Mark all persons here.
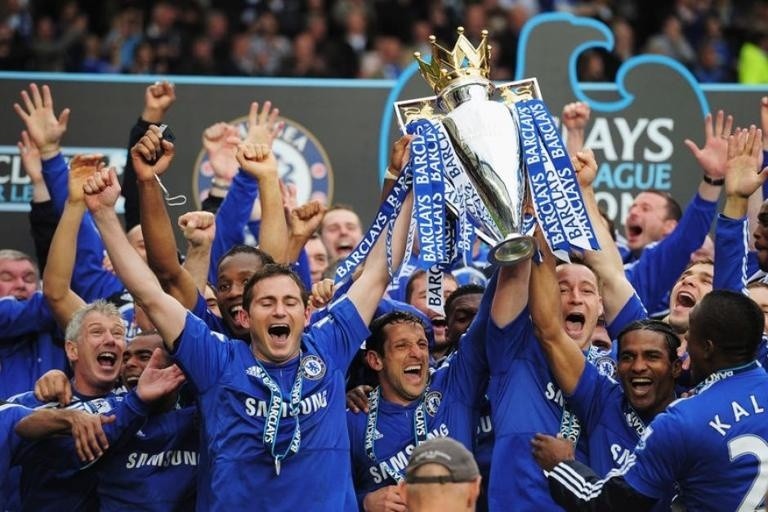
[0,0,767,511]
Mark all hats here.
[405,437,480,484]
